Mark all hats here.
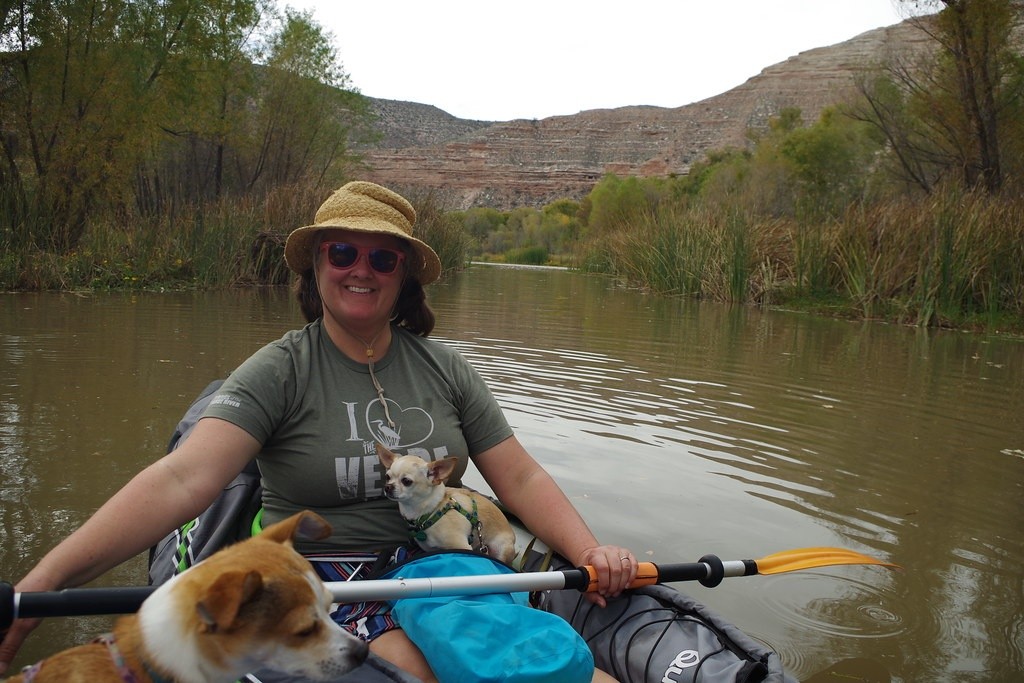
[284,181,441,286]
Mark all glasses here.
[318,242,407,275]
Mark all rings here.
[620,556,629,561]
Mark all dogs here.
[0,510,370,683]
[374,442,516,567]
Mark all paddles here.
[0,548,902,643]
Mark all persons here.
[0,181,639,683]
